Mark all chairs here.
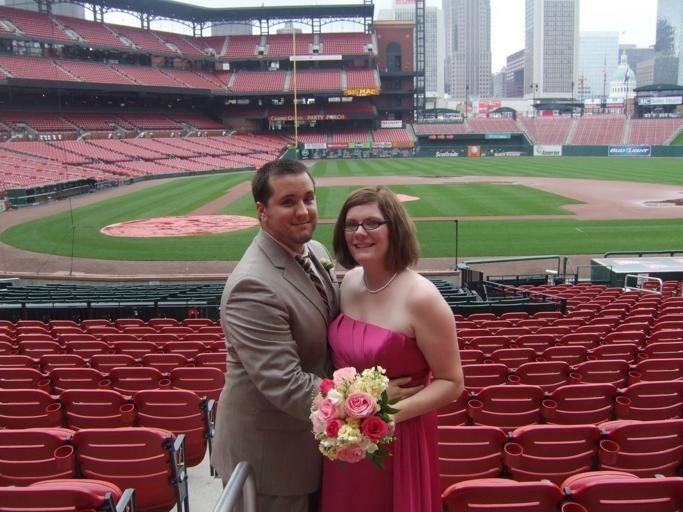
[1,278,682,512]
[0,7,683,202]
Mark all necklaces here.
[361,268,401,293]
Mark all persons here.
[309,184,465,511]
[211,161,423,512]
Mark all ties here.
[302,256,331,315]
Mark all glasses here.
[344,219,388,230]
